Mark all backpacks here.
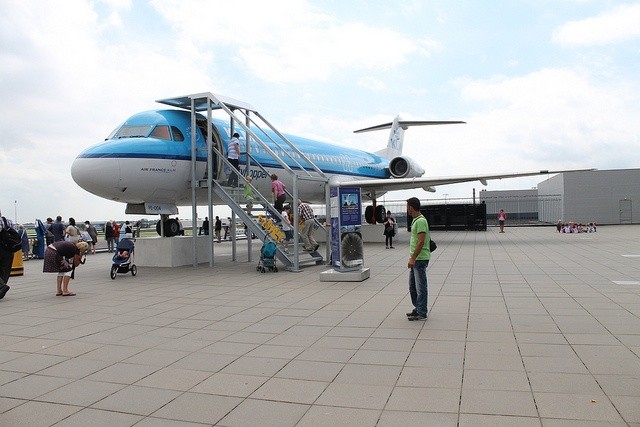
[0,217,22,253]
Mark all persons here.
[0,277,9,298]
[564,223,570,232]
[557,219,562,231]
[44,217,54,247]
[119,250,128,256]
[0,216,22,284]
[570,225,575,232]
[112,221,120,246]
[227,132,240,187]
[50,215,66,242]
[124,221,132,237]
[105,221,114,252]
[215,215,222,242]
[588,222,594,232]
[203,216,209,235]
[562,223,565,232]
[243,221,248,236]
[43,240,90,296]
[406,197,437,320]
[383,209,396,248]
[281,204,291,240]
[132,219,142,236]
[31,240,40,259]
[296,198,320,251]
[577,223,587,232]
[497,207,506,232]
[270,173,287,213]
[227,216,232,234]
[66,216,80,238]
[587,223,596,231]
[35,218,48,258]
[18,224,30,260]
[84,220,97,254]
[243,175,254,215]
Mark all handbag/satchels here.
[430,239,437,252]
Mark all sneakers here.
[407,309,419,316]
[0,285,9,299]
[390,247,395,249]
[314,244,319,251]
[408,314,427,321]
[386,247,389,249]
[303,248,313,251]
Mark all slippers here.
[63,291,76,296]
[56,293,62,296]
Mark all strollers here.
[111,238,137,279]
[257,231,278,273]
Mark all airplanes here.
[71,109,597,238]
